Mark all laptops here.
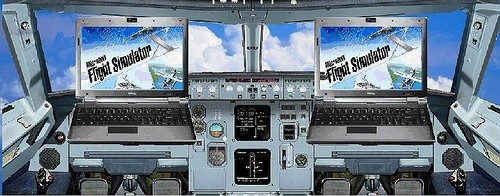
[67,16,197,146]
[305,15,436,146]
